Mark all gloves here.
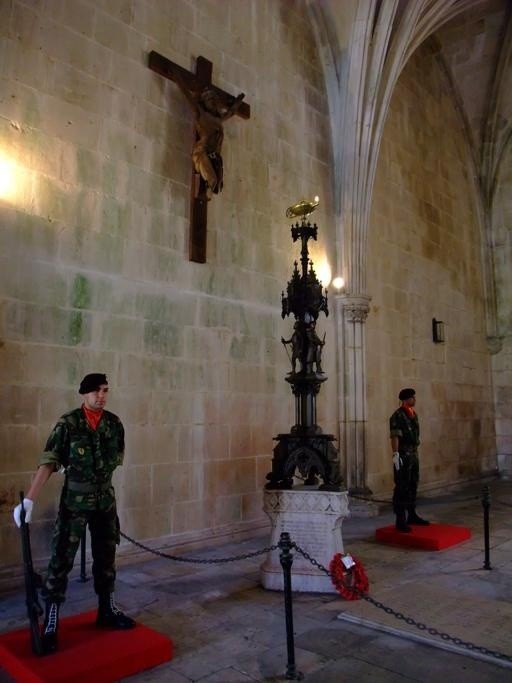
[14,498,34,528]
[392,452,404,470]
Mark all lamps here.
[433,317,445,342]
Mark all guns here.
[393,448,406,530]
[19,490,43,657]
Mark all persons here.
[12,372,137,651]
[389,386,430,532]
[175,72,244,201]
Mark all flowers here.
[329,554,370,602]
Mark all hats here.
[78,374,108,395]
[399,389,415,399]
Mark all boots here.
[395,506,429,533]
[95,592,135,631]
[41,602,60,654]
